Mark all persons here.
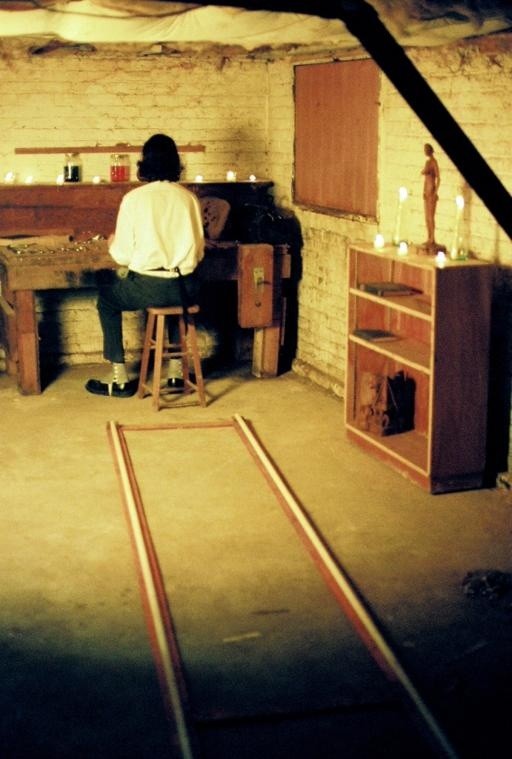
[420,144,440,244]
[86,134,206,397]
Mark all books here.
[354,329,397,343]
[359,281,411,296]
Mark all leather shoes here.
[85,378,132,397]
[168,373,195,396]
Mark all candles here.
[193,170,260,183]
[435,251,445,265]
[2,169,100,186]
[373,233,386,247]
[395,241,408,257]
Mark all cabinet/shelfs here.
[343,238,496,496]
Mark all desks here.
[1,240,294,392]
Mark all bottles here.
[64,150,84,182]
[108,153,132,182]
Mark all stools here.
[138,301,209,409]
